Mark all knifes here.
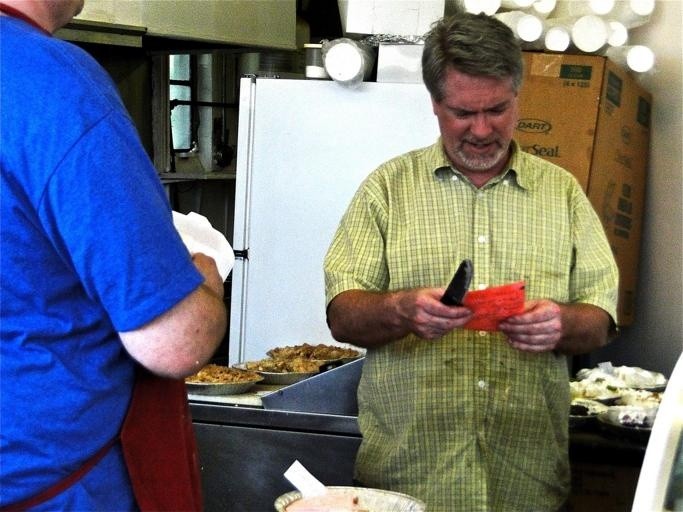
[438,259,473,307]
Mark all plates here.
[274,487,425,512]
[568,368,665,434]
[229,359,313,386]
[264,350,360,362]
[184,382,254,398]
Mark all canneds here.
[303,44,327,79]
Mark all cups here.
[301,43,328,79]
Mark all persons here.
[323,11,622,512]
[0,2,240,511]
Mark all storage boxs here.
[510,51,653,330]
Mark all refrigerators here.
[222,78,440,364]
